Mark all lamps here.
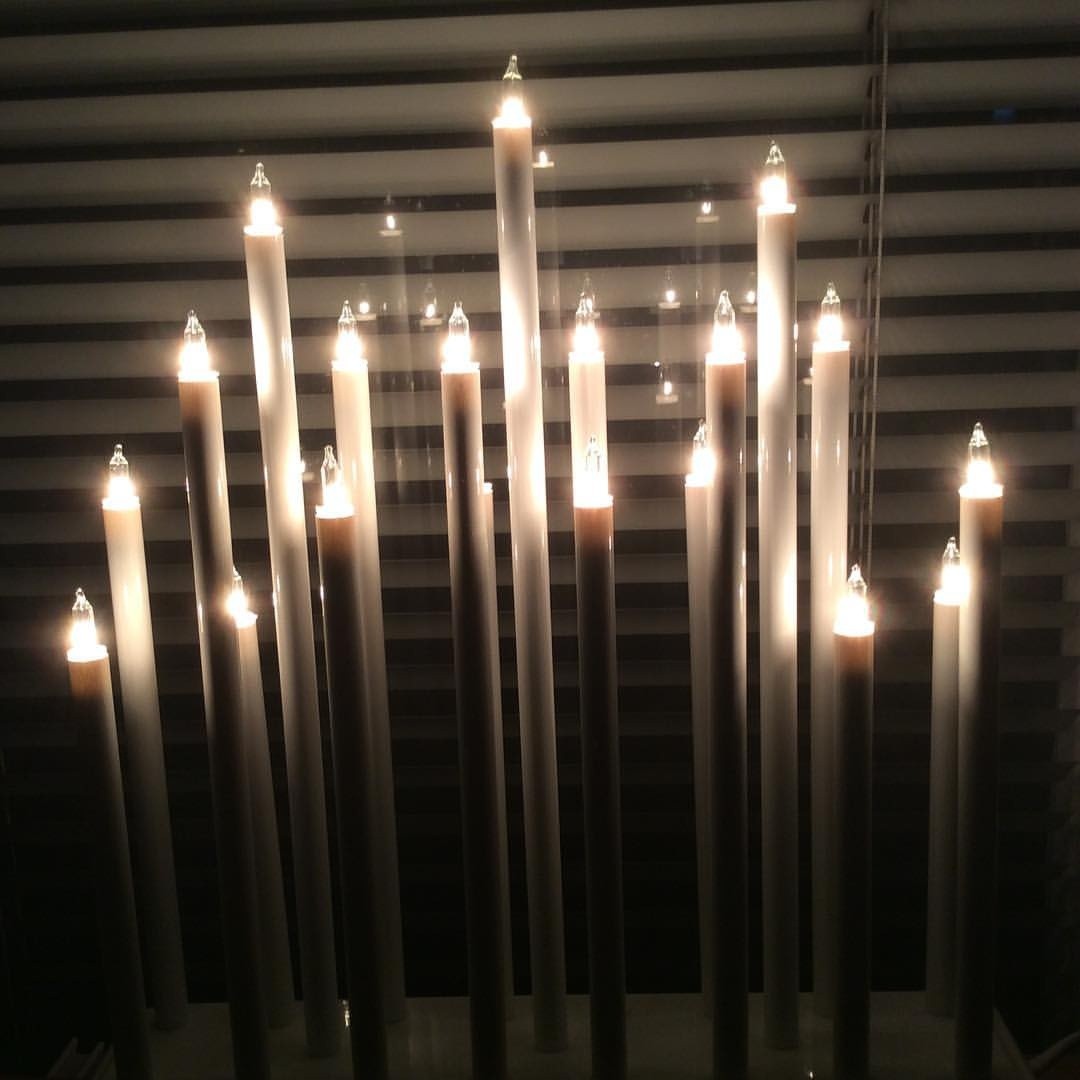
[64,53,1003,1080]
